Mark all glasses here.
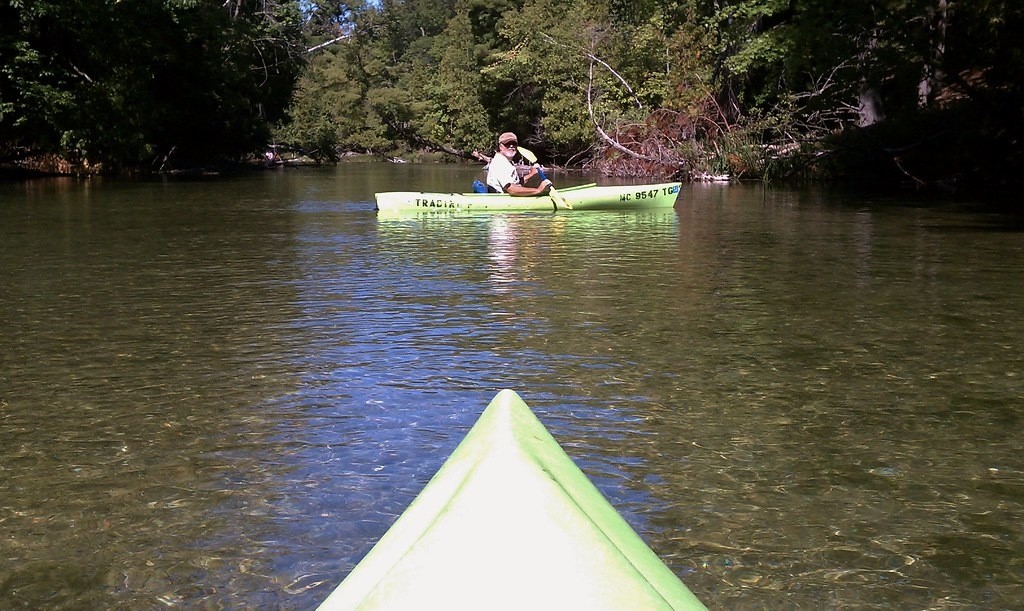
[503,142,516,147]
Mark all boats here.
[373,182,684,214]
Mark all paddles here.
[517,145,573,210]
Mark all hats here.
[498,131,518,146]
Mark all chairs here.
[473,180,487,193]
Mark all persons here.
[486,132,553,197]
